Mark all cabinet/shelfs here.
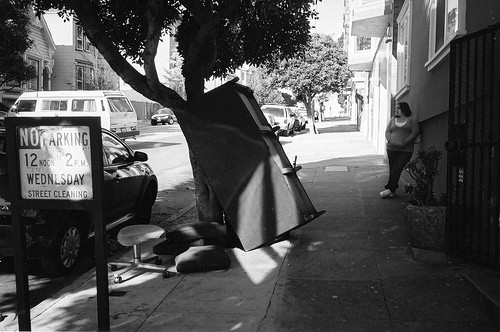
[175,76,327,252]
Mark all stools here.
[108,224,180,283]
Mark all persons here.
[380,101,421,197]
[320,102,326,122]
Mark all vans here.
[6,91,141,140]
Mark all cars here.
[259,103,310,139]
[0,129,159,276]
[151,108,175,126]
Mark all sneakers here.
[389,192,396,198]
[379,189,391,197]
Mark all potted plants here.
[403,148,446,250]
